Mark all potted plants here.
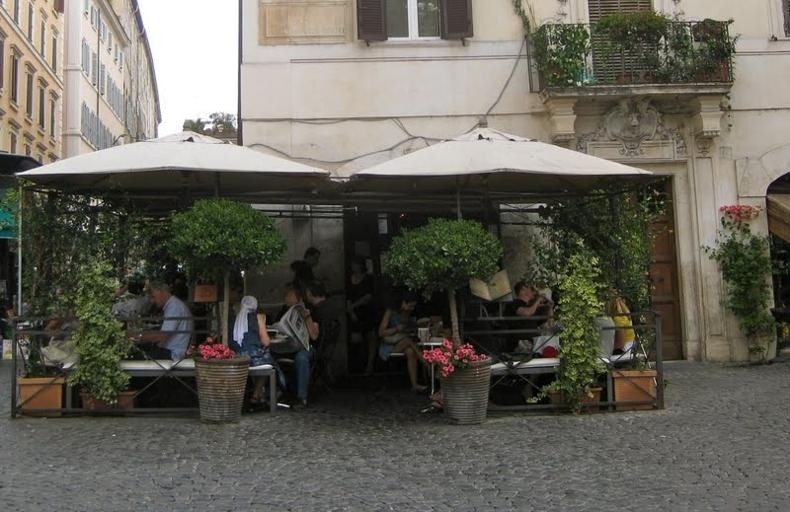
[526,227,603,413]
[378,216,500,425]
[13,188,73,419]
[547,185,658,411]
[69,255,137,412]
[166,196,287,423]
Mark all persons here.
[41,247,346,415]
[346,260,635,415]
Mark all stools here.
[389,352,406,356]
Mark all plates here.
[271,335,289,344]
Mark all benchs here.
[490,353,644,411]
[63,358,278,418]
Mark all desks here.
[270,335,288,346]
[468,314,546,351]
[126,330,165,360]
[417,336,450,415]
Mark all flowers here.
[182,342,233,361]
[423,339,486,379]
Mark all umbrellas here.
[12,129,330,204]
[350,127,651,226]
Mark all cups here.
[417,327,431,343]
